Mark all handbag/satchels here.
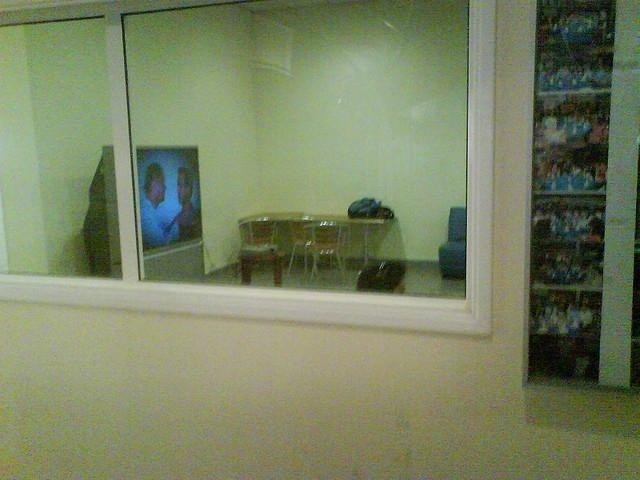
[348,199,394,219]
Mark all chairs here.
[236,215,349,289]
[437,206,467,280]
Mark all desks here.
[238,212,387,269]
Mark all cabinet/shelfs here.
[529,1,640,395]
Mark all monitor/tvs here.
[81,145,205,266]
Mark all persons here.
[169,167,199,236]
[355,262,408,296]
[136,163,180,245]
